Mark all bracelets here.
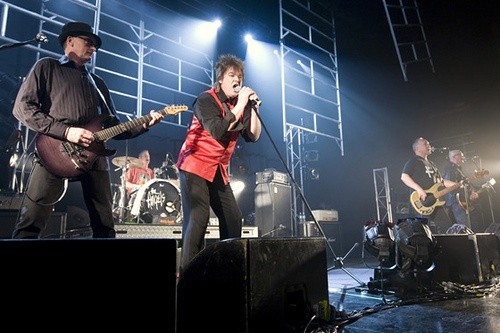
[64,126,70,139]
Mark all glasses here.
[78,36,99,52]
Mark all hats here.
[58,21,103,49]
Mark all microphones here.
[35,32,49,44]
[164,153,169,166]
[235,86,263,106]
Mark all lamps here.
[363,220,436,274]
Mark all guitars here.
[34,103,189,179]
[410,169,489,216]
[457,178,496,212]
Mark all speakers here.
[175,237,327,332]
[408,232,500,286]
[0,238,176,333]
[254,183,293,237]
[0,210,68,239]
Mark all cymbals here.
[111,157,144,168]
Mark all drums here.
[156,166,180,188]
[137,181,182,225]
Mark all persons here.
[441,149,478,230]
[13,22,164,239]
[401,137,467,234]
[125,151,154,223]
[176,55,262,277]
